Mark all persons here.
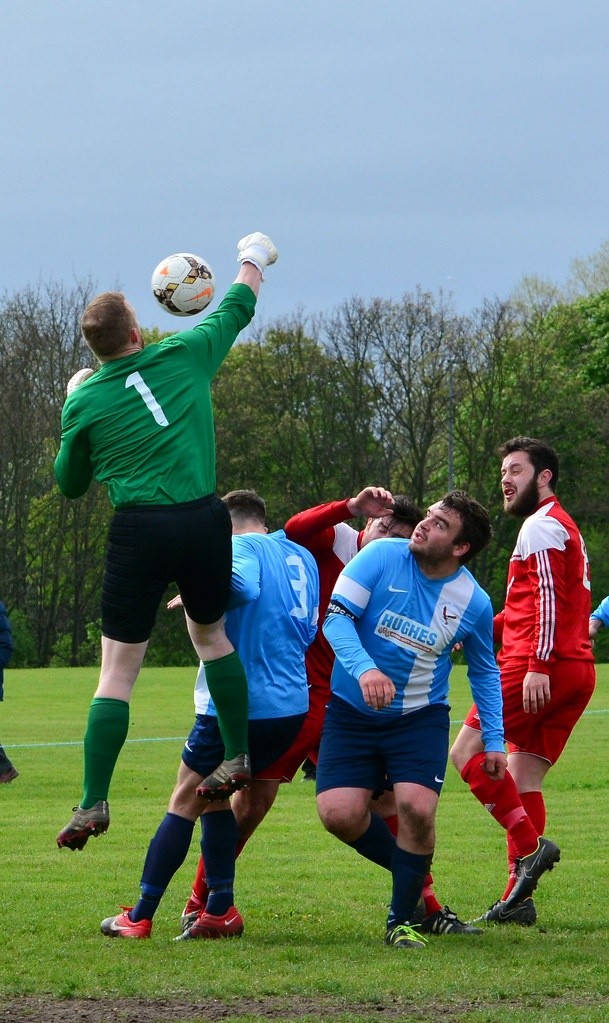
[313,490,506,949]
[447,437,597,924]
[178,485,490,937]
[53,229,281,851]
[94,490,318,937]
[0,600,19,785]
[588,596,609,646]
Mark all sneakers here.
[56,801,109,853]
[181,905,204,933]
[384,924,428,951]
[100,906,154,941]
[0,767,19,783]
[465,893,537,925]
[413,905,485,937]
[170,906,244,940]
[195,755,253,799]
[504,836,561,908]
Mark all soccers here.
[152,252,215,316]
